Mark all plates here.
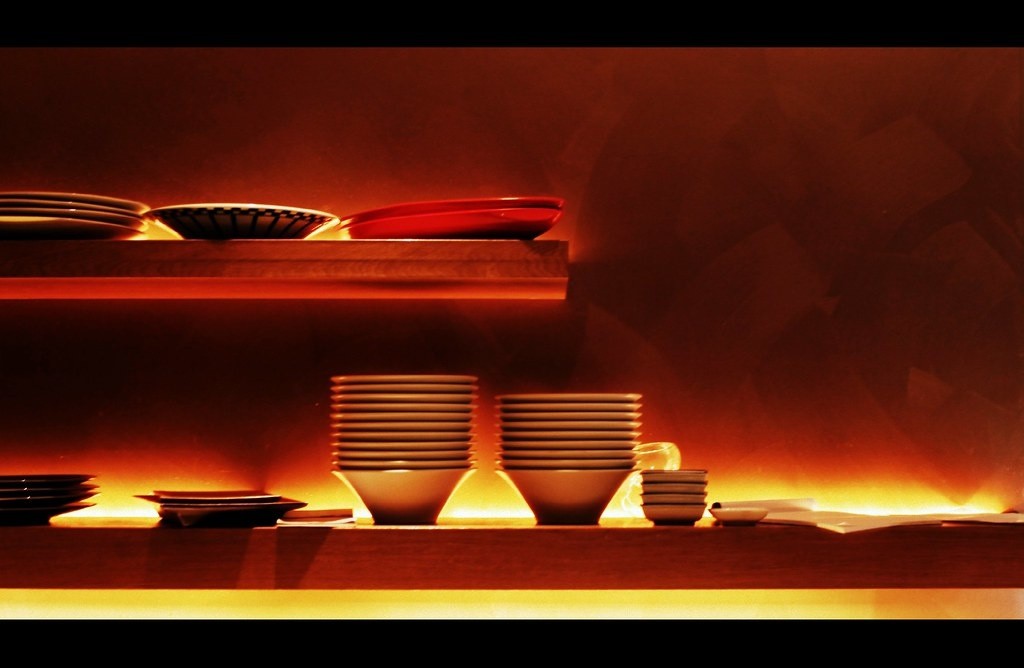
[329,375,480,525]
[0,189,149,240]
[140,202,340,239]
[708,508,769,526]
[338,196,566,239]
[0,472,102,527]
[133,491,308,528]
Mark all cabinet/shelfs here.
[1,236,1024,588]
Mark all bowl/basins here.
[493,394,642,526]
[640,470,708,526]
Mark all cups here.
[634,442,681,485]
